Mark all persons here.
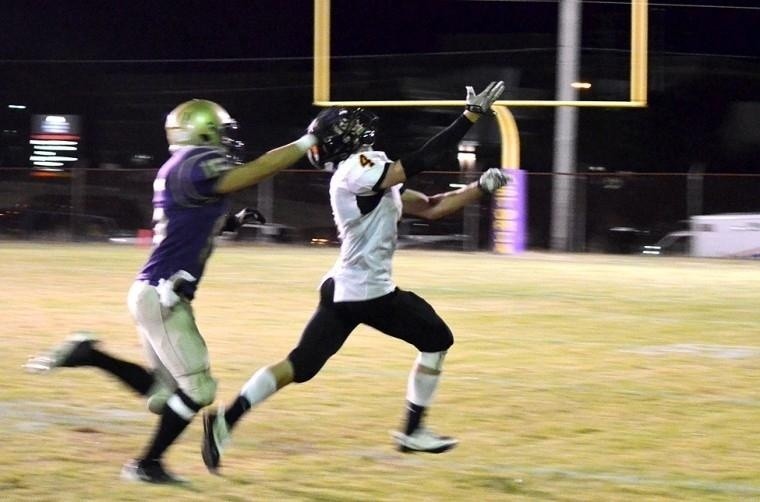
[18,97,348,492]
[200,79,510,475]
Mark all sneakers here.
[389,428,458,453]
[202,403,227,469]
[23,333,101,374]
[122,454,190,484]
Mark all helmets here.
[164,98,246,165]
[307,106,380,172]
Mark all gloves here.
[479,168,512,195]
[223,207,265,231]
[465,80,506,116]
[307,107,348,146]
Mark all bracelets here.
[294,131,321,155]
